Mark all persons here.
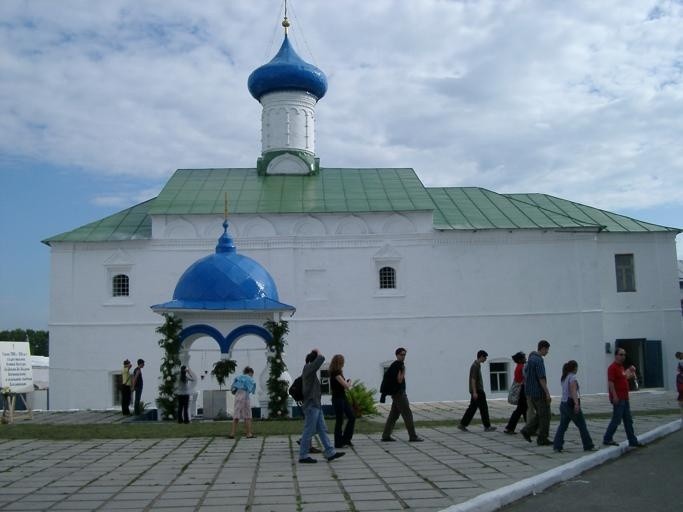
[174,365,197,424]
[298,348,346,463]
[504,351,527,434]
[520,340,553,446]
[121,359,133,416]
[329,354,356,448]
[381,348,424,441]
[296,354,322,453]
[603,347,647,448]
[675,352,683,402]
[131,359,145,416]
[553,360,600,453]
[226,366,256,438]
[457,350,497,432]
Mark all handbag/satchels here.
[289,376,305,402]
[508,382,522,406]
[567,397,581,408]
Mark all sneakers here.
[484,427,496,432]
[590,446,601,452]
[457,423,469,432]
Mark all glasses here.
[618,354,628,357]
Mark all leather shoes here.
[310,447,322,454]
[603,440,618,446]
[629,442,647,448]
[520,430,533,443]
[299,458,318,464]
[329,452,346,461]
[537,439,554,446]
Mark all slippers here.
[381,437,397,441]
[409,438,424,441]
[504,429,517,434]
